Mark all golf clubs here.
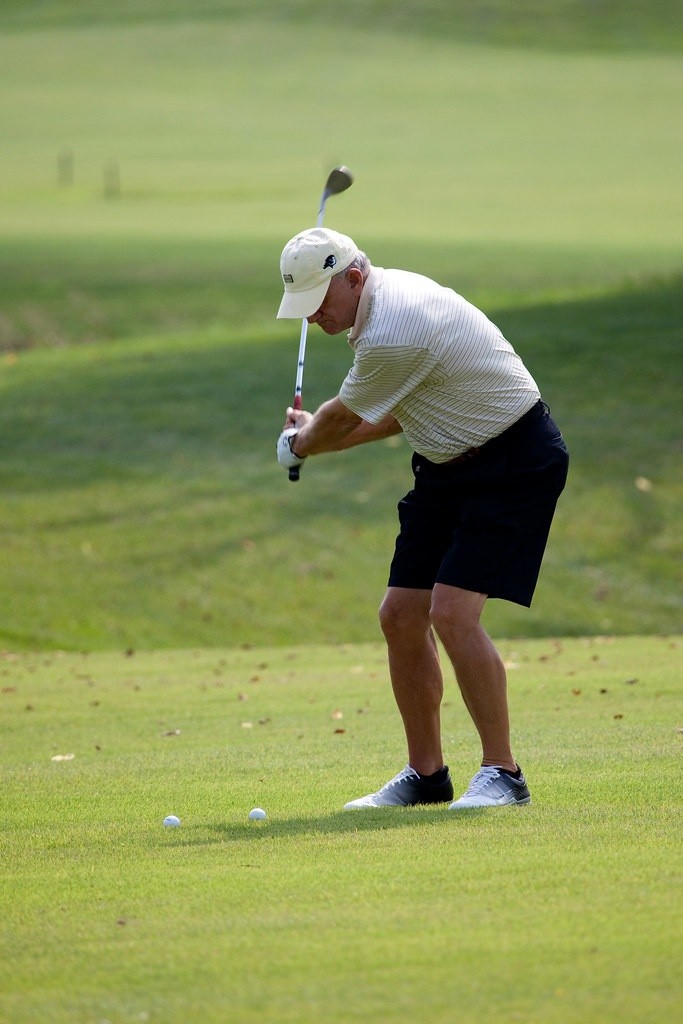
[288,166,355,482]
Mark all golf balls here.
[163,815,180,828]
[249,808,266,819]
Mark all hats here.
[276,225,359,319]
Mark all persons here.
[276,229,570,814]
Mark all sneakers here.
[343,763,454,810]
[448,763,532,808]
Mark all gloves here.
[276,428,308,469]
